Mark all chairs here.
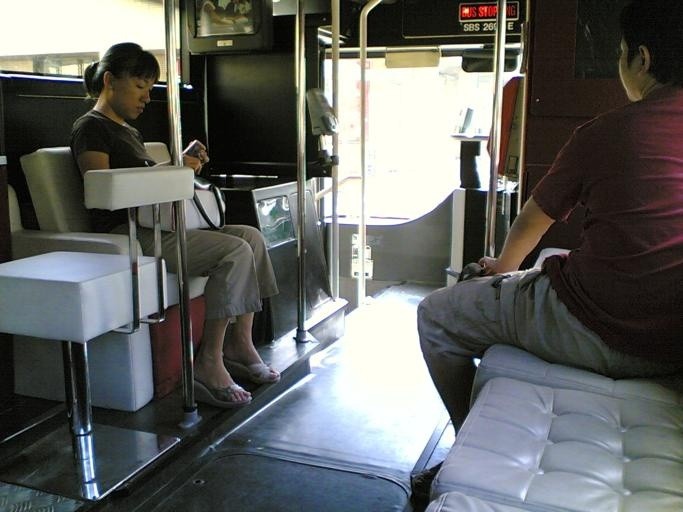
[9,145,211,412]
[425,248,683,512]
[0,165,195,503]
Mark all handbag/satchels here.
[138,157,227,231]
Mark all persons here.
[418,1,683,436]
[70,42,281,409]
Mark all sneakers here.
[409,456,448,499]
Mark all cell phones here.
[182,140,207,160]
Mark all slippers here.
[221,352,282,386]
[181,373,254,409]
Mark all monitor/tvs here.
[187,0,274,55]
